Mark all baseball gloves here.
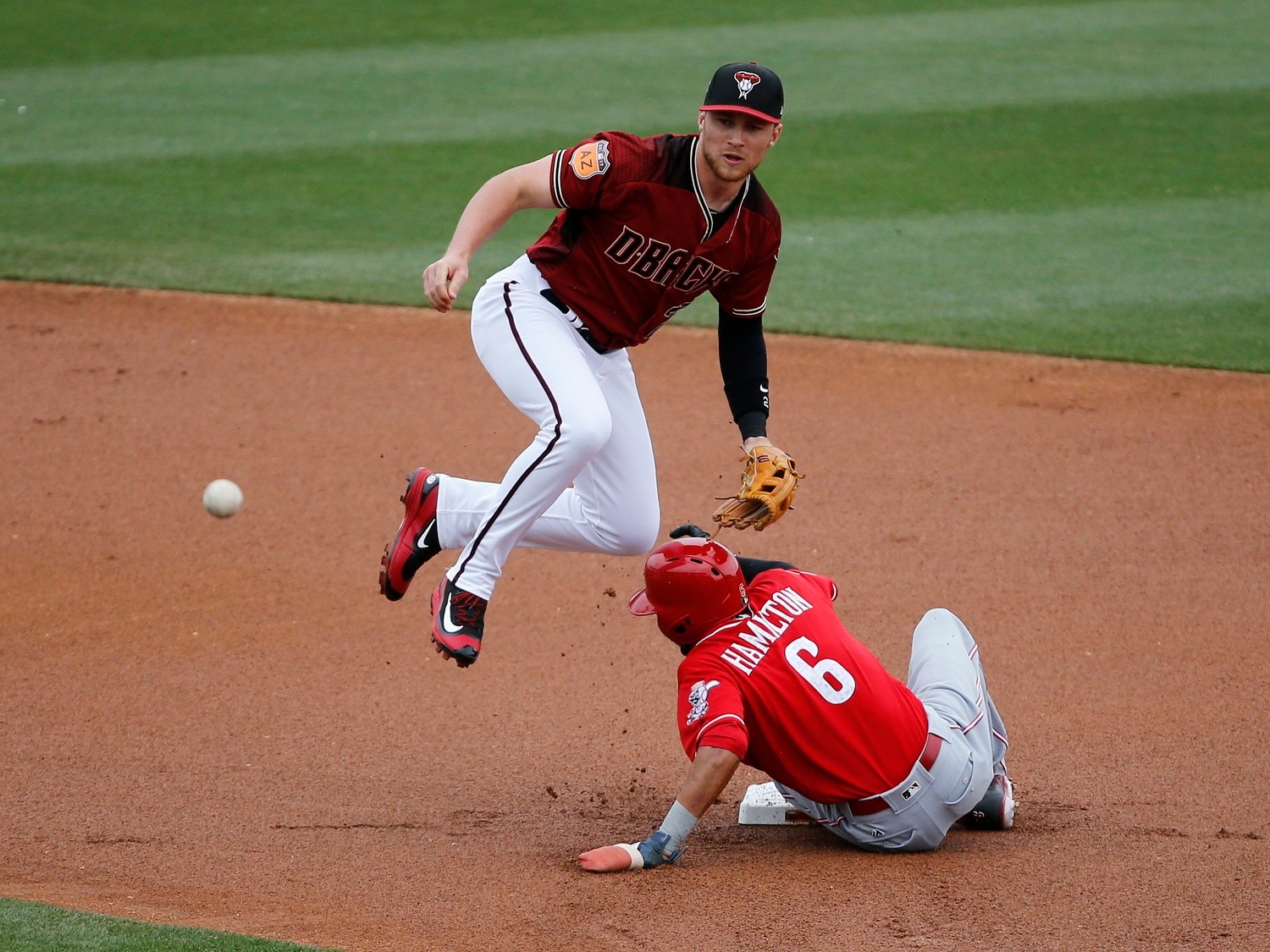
[705,444,806,544]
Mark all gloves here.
[579,841,665,874]
[668,526,711,542]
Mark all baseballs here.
[201,479,244,520]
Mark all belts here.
[541,289,617,355]
[837,732,941,819]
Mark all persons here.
[578,524,1015,872]
[379,61,797,669]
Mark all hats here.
[700,61,786,125]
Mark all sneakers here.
[377,466,442,602]
[954,772,1013,832]
[430,576,488,669]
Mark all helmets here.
[629,535,748,645]
